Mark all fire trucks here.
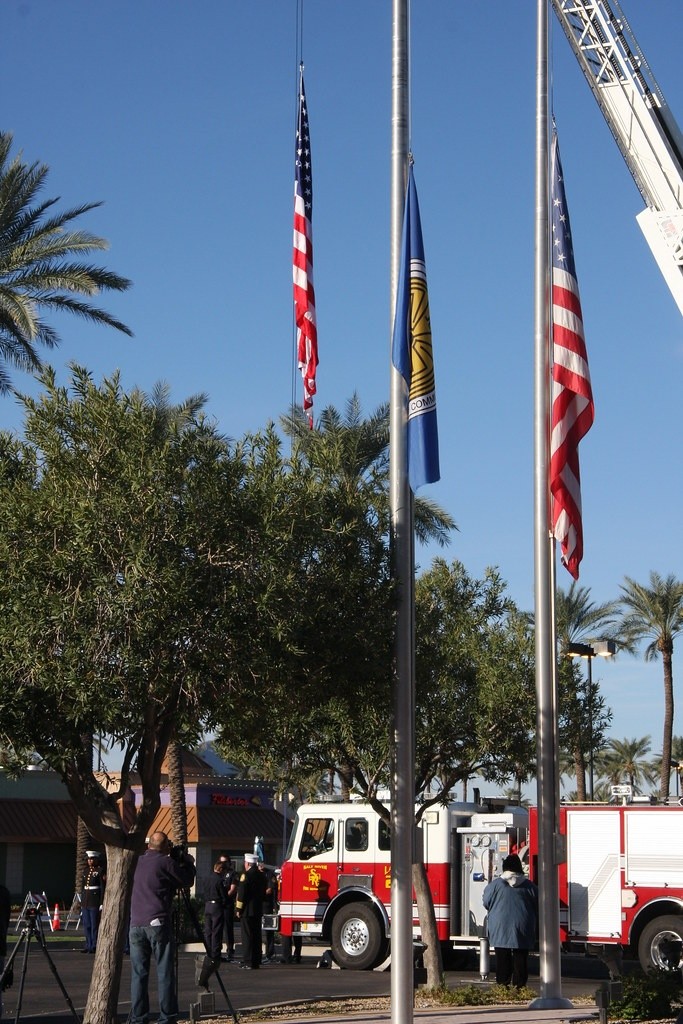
[262,0,683,984]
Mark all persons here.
[257,865,278,965]
[219,854,237,962]
[129,832,195,1024]
[238,853,265,970]
[302,835,334,854]
[482,854,538,991]
[80,851,104,953]
[276,935,302,964]
[196,861,228,990]
[352,823,368,851]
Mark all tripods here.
[0,908,82,1024]
[174,886,240,1024]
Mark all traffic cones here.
[49,904,64,931]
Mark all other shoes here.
[80,949,96,954]
[224,954,235,961]
[276,958,290,965]
[261,957,273,964]
[237,964,252,969]
[291,956,302,964]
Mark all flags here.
[391,156,441,498]
[292,66,321,432]
[549,127,596,582]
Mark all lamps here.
[413,939,429,969]
[193,953,221,991]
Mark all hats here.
[244,853,259,864]
[85,850,101,858]
[503,854,523,874]
